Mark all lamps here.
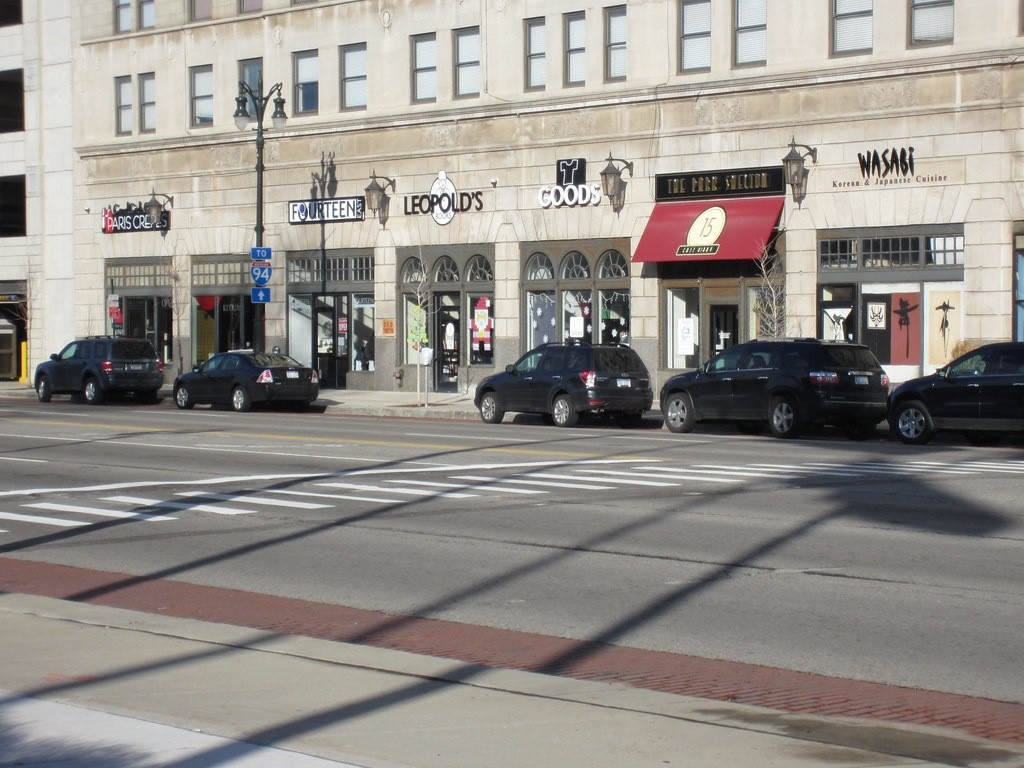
[146,186,174,231]
[781,134,817,187]
[364,169,396,219]
[600,149,633,202]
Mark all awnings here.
[630,196,784,262]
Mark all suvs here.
[35,335,163,405]
[473,342,653,427]
[660,335,889,439]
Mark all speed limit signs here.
[251,262,273,286]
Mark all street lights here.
[234,70,288,353]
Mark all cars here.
[888,341,1024,445]
[173,350,318,412]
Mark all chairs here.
[999,358,1019,374]
[751,355,766,369]
